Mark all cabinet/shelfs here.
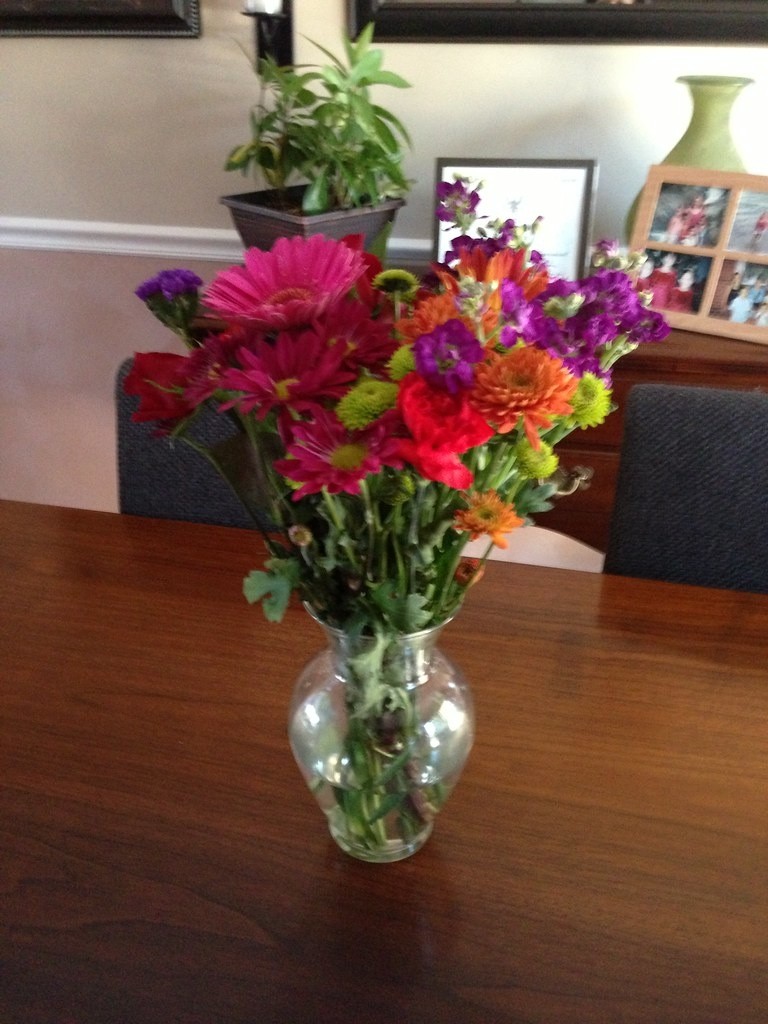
[528,319,768,551]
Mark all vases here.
[624,74,755,244]
[281,601,479,861]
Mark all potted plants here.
[216,25,413,261]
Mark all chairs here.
[604,386,768,589]
[116,354,289,529]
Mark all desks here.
[0,501,768,1022]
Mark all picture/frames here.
[347,0,768,40]
[609,165,768,346]
[0,0,203,40]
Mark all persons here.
[634,194,768,328]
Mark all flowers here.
[117,174,678,653]
